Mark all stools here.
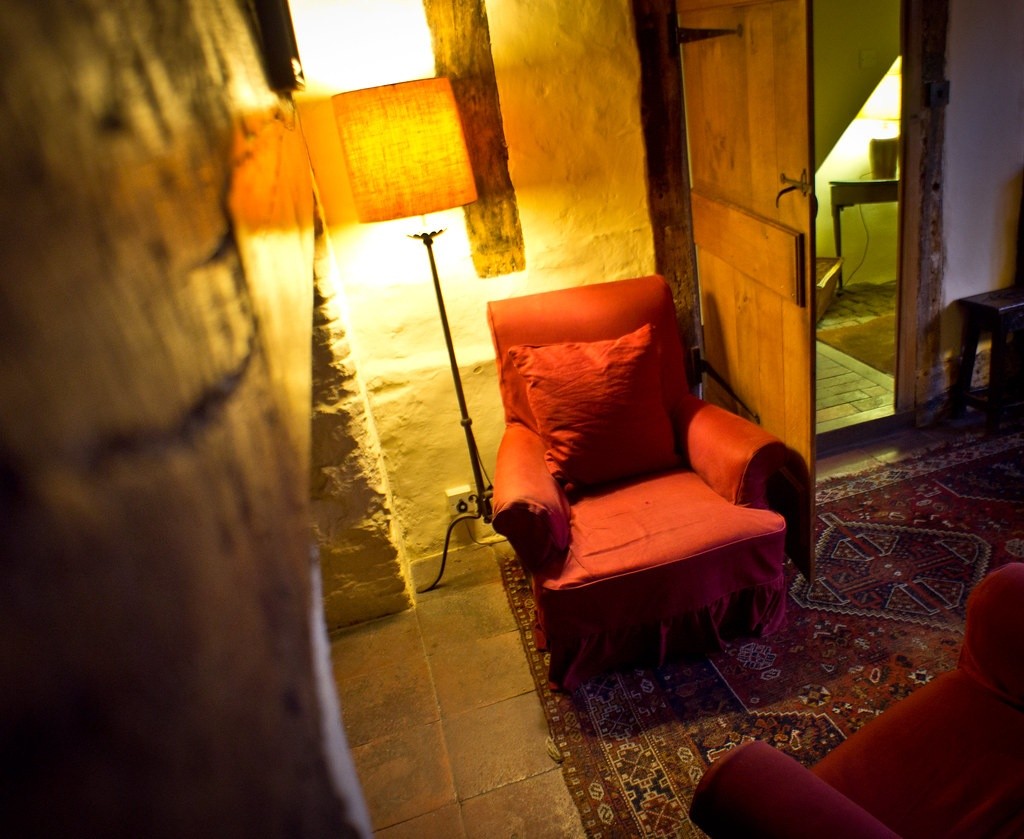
[948,285,1024,417]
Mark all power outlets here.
[445,484,477,517]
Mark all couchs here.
[684,563,1024,839]
[487,274,810,708]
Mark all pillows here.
[506,322,678,495]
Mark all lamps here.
[865,72,902,177]
[332,77,510,596]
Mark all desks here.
[829,177,902,291]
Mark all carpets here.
[496,422,1024,839]
